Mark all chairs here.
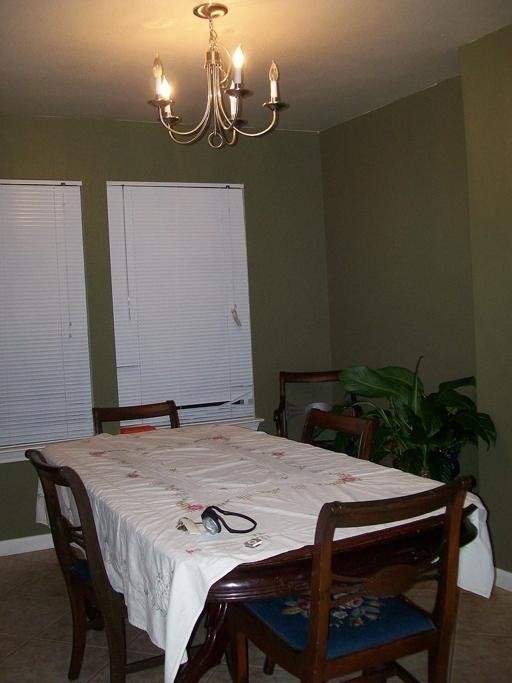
[300,407,374,461]
[25,447,213,681]
[238,474,473,680]
[90,399,181,436]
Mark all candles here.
[147,1,287,148]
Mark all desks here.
[31,422,496,682]
